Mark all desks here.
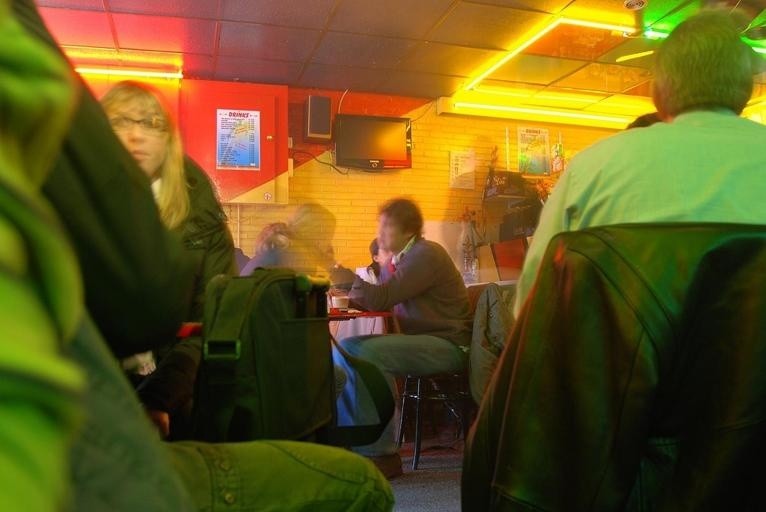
[330,309,393,321]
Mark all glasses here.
[111,117,163,132]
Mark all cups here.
[332,296,349,312]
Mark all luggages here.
[194,267,331,442]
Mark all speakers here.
[303,95,333,140]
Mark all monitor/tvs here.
[335,113,412,172]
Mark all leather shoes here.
[369,454,403,479]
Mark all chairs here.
[393,282,499,472]
[651,236,766,512]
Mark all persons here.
[1,0,766,512]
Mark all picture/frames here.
[518,127,551,177]
[448,150,476,190]
